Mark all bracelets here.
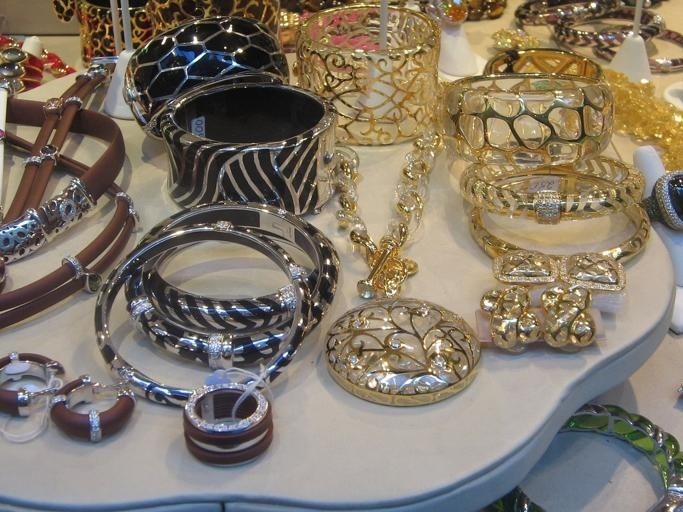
[2,3,682,512]
